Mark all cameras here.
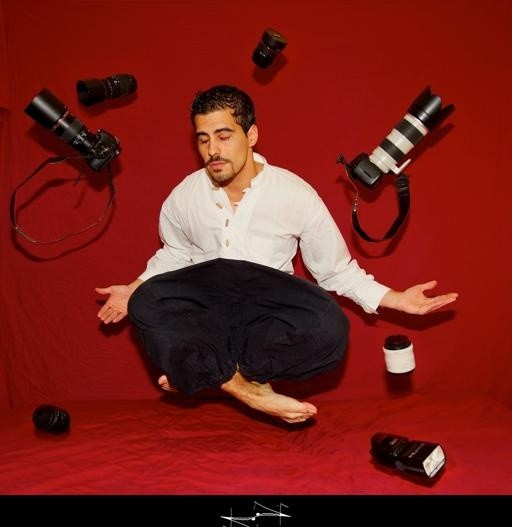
[24,88,121,171]
[349,85,455,189]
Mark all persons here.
[95,86,459,426]
